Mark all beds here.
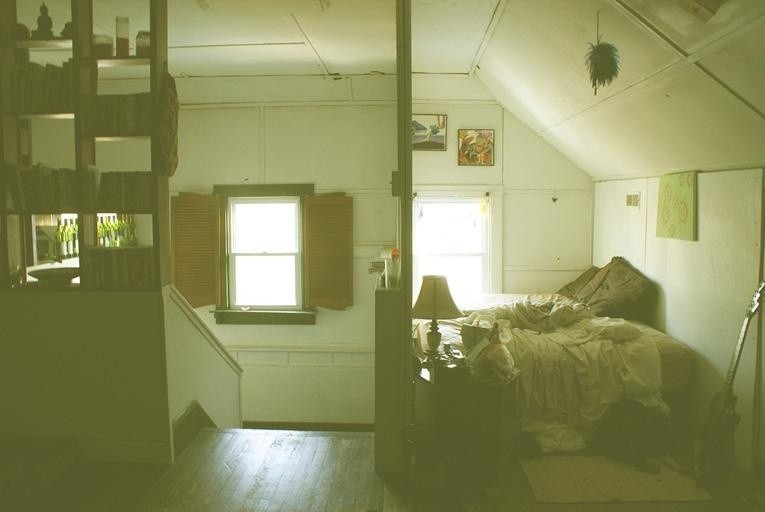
[412,287,696,445]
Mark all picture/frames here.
[457,127,496,168]
[411,113,450,152]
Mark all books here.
[88,170,152,210]
[7,58,73,111]
[6,161,76,210]
[93,92,150,132]
[85,244,155,290]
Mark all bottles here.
[94,16,150,59]
[55,212,136,258]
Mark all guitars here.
[687,281,765,500]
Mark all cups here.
[385,260,400,288]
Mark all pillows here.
[556,258,658,317]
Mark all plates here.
[28,268,80,284]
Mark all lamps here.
[409,276,469,366]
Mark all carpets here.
[520,455,714,504]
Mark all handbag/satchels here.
[462,316,498,347]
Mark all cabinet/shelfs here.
[375,274,407,473]
[0,0,174,291]
